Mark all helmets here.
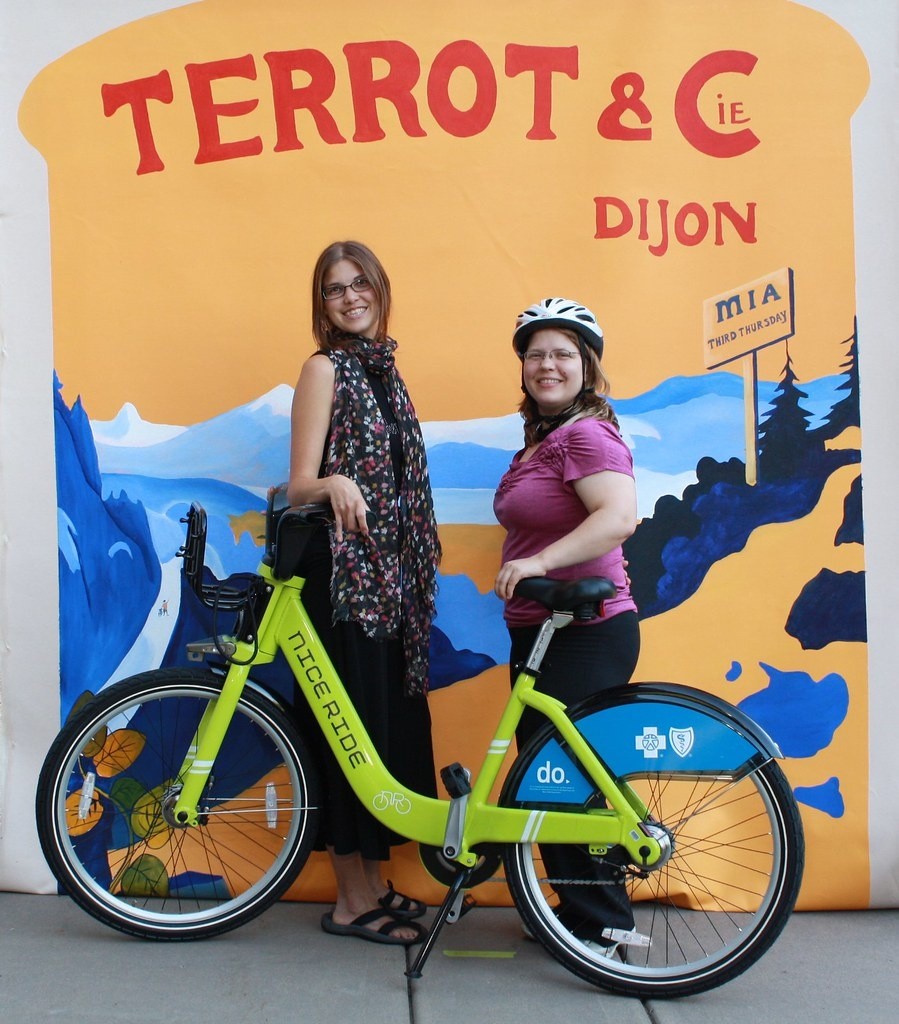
[510,297,604,359]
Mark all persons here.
[494,303,642,959]
[286,241,436,943]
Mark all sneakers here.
[580,923,636,958]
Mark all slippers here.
[319,877,430,946]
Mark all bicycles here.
[31,485,806,1002]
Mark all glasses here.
[521,348,581,361]
[321,278,373,300]
